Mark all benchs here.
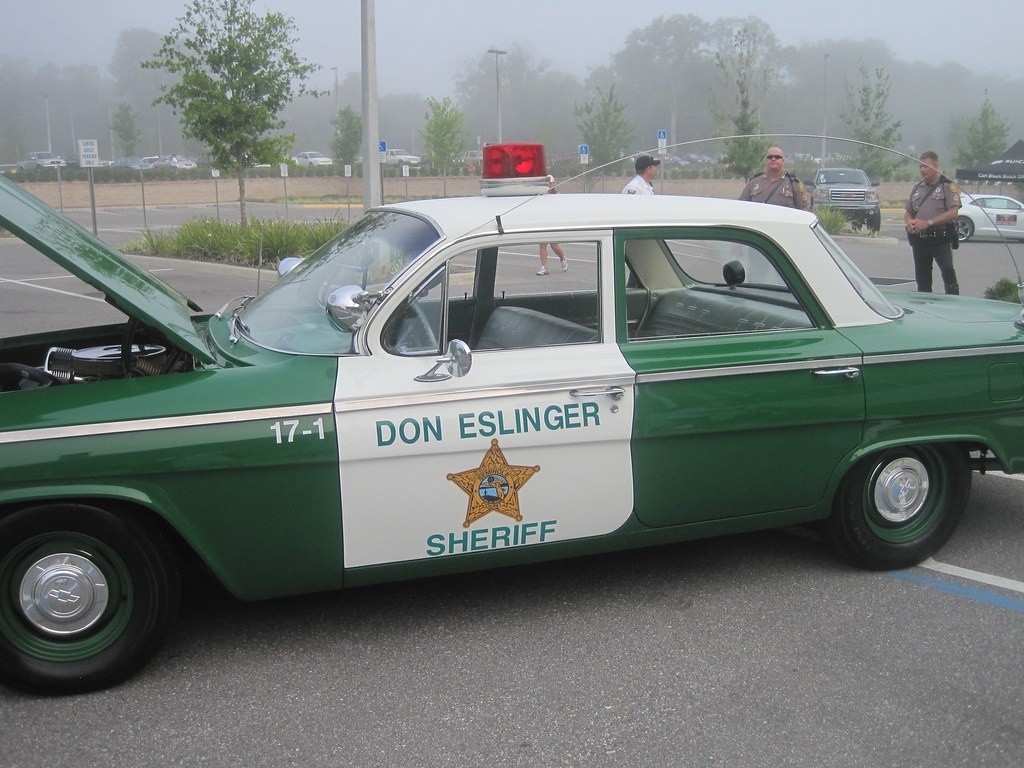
[637,287,816,337]
[475,306,599,347]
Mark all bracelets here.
[928,219,933,225]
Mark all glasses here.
[767,154,783,160]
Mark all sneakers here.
[560,256,568,271]
[536,266,550,275]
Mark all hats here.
[548,174,555,184]
[635,155,661,170]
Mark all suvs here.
[797,166,881,235]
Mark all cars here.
[952,194,1024,240]
[0,140,857,190]
[0,176,1024,691]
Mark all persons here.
[904,150,962,297]
[736,146,808,212]
[622,152,660,288]
[537,174,569,275]
